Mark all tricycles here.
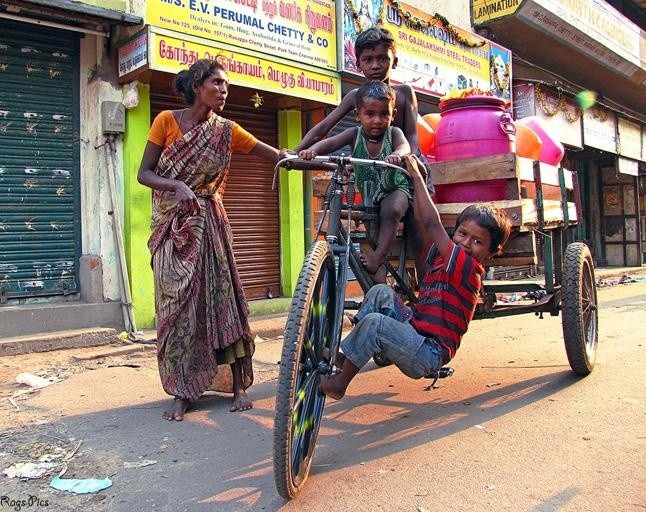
[268,84,600,500]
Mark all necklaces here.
[363,132,385,144]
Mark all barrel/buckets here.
[417,95,565,203]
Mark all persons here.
[353,0,373,34]
[317,153,513,401]
[134,57,357,423]
[296,79,414,286]
[277,25,437,292]
